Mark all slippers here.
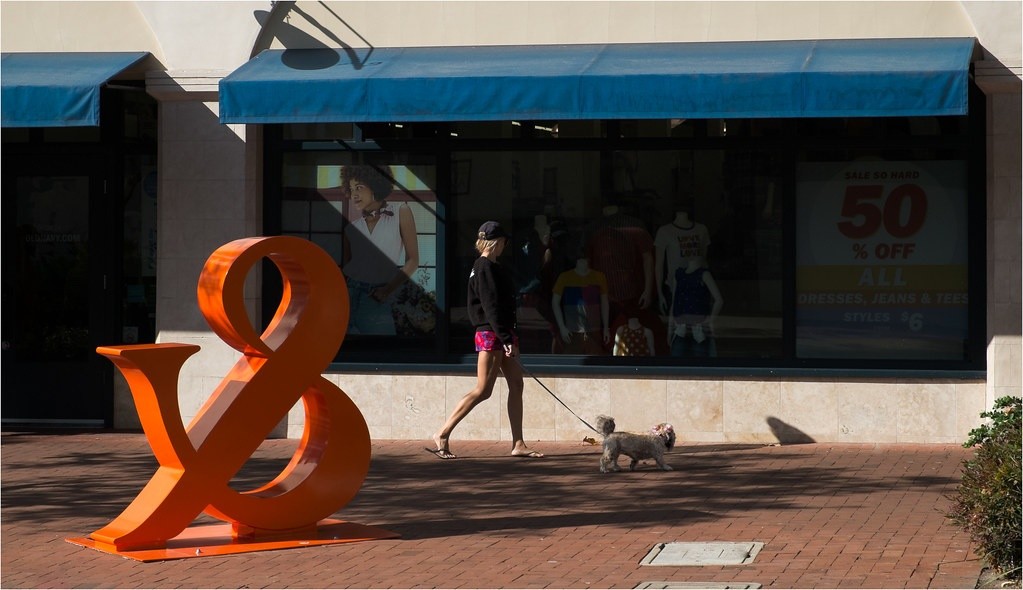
[513,451,544,458]
[425,447,449,459]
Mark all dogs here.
[595,414,676,474]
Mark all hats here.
[477,221,511,240]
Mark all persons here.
[333,150,419,334]
[552,260,611,354]
[433,221,546,458]
[537,228,555,292]
[587,203,726,357]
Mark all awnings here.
[1,52,150,127]
[219,35,981,126]
